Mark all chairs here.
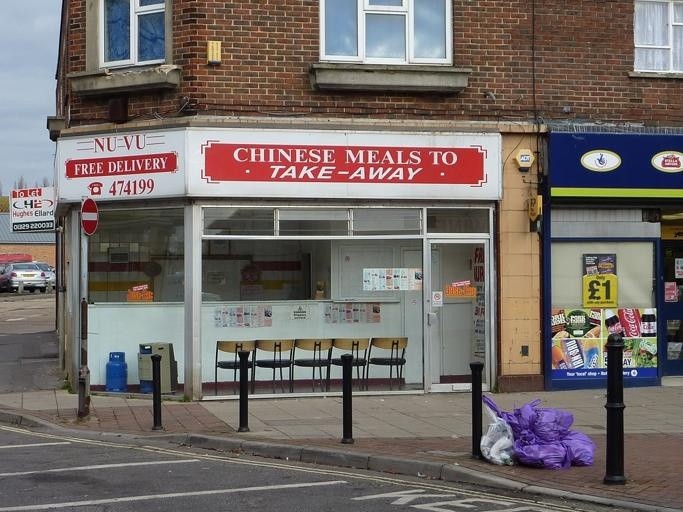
[213,335,411,394]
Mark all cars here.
[0,262,47,295]
[33,260,56,291]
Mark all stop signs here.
[80,196,99,237]
